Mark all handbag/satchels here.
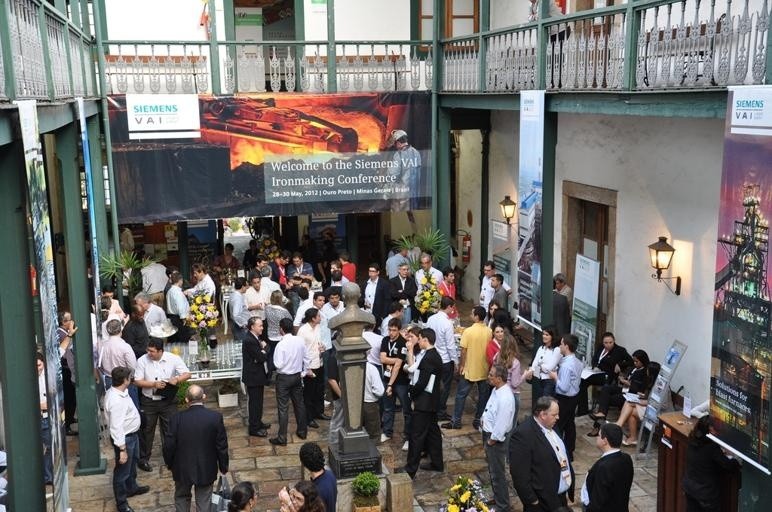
[211,476,232,512]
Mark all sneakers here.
[380,433,409,451]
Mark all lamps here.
[648,237,681,296]
[500,195,517,225]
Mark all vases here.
[351,471,384,512]
[198,348,211,369]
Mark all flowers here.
[184,289,219,344]
[439,475,496,512]
[414,268,440,317]
[258,237,282,267]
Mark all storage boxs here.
[218,389,238,407]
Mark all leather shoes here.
[118,502,134,512]
[269,438,286,446]
[296,414,331,439]
[127,486,149,497]
[420,463,443,472]
[65,418,78,435]
[622,440,637,445]
[138,461,152,471]
[249,424,270,437]
[587,414,605,437]
[438,414,461,429]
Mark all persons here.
[691,401,709,419]
[386,130,421,211]
[0,232,676,511]
[681,416,743,512]
